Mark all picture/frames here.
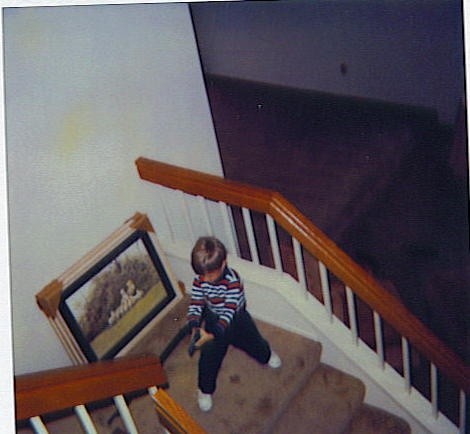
[35,212,186,366]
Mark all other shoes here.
[198,389,212,411]
[268,351,281,369]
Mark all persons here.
[187,236,282,412]
